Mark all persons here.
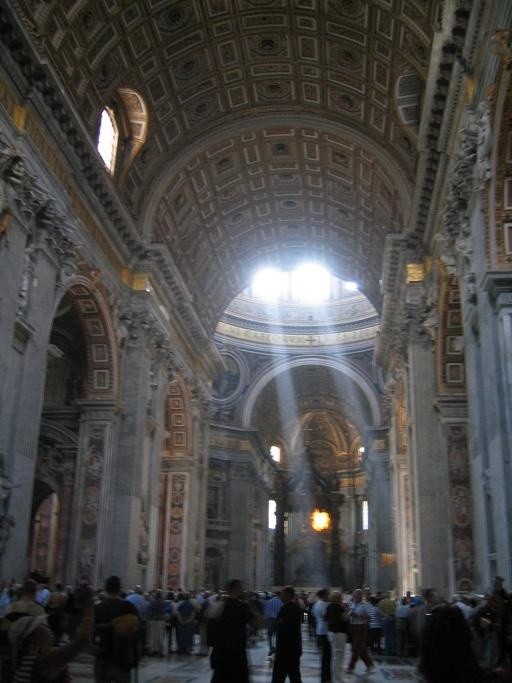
[0,572,512,683]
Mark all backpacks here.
[107,614,147,668]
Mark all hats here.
[8,613,51,646]
